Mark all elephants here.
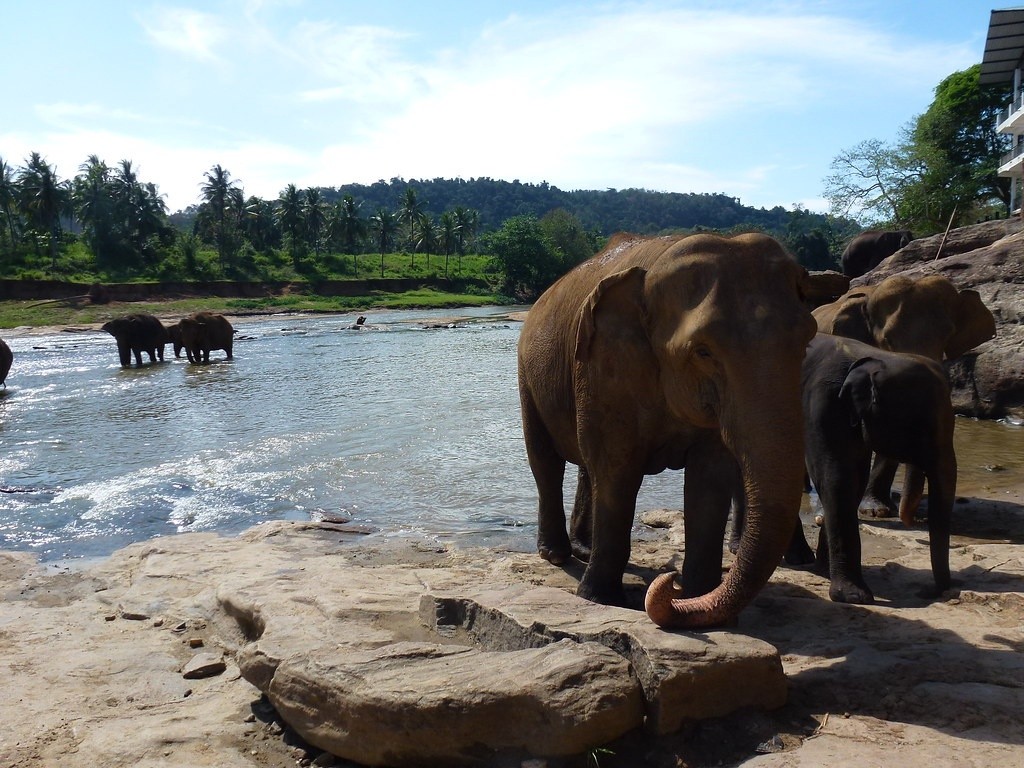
[0,337,14,390]
[100,310,239,367]
[515,227,996,629]
